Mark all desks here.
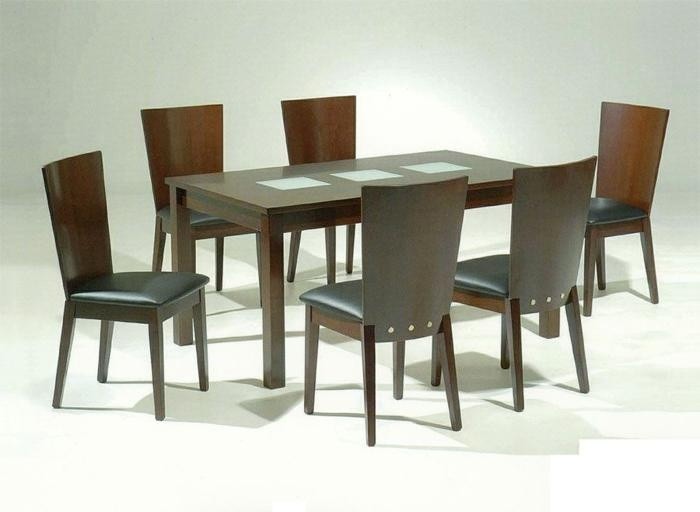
[165,149,537,390]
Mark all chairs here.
[431,156,599,412]
[280,95,358,282]
[141,103,265,307]
[42,151,211,422]
[583,102,670,316]
[298,175,470,445]
